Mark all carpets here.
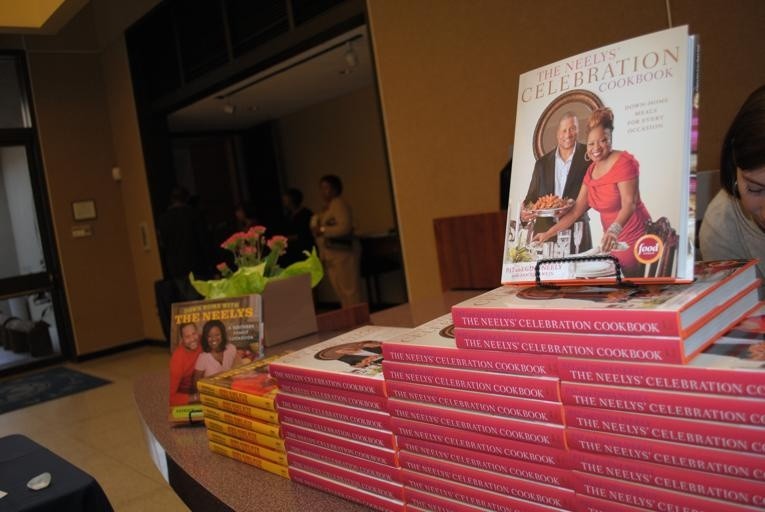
[0,361,117,416]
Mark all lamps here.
[345,42,357,66]
[222,100,235,113]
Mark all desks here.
[135,290,765,512]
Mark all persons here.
[336,341,384,368]
[234,200,262,232]
[310,174,360,309]
[518,111,594,255]
[157,189,211,300]
[171,321,202,405]
[285,189,319,266]
[191,320,243,392]
[531,109,656,277]
[699,85,765,302]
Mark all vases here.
[257,272,321,348]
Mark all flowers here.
[188,225,325,304]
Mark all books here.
[278,409,397,451]
[405,505,431,512]
[561,382,765,428]
[288,464,405,512]
[566,425,765,481]
[564,406,765,455]
[277,391,394,433]
[451,258,761,335]
[388,397,566,449]
[557,302,765,397]
[204,406,283,439]
[400,466,572,512]
[501,24,702,284]
[271,326,416,397]
[395,436,575,490]
[390,416,570,469]
[206,438,288,479]
[386,380,564,425]
[169,294,265,426]
[282,423,399,468]
[287,453,405,502]
[570,448,765,510]
[382,360,561,404]
[197,349,297,411]
[206,430,288,465]
[286,438,401,484]
[574,490,657,512]
[275,379,390,414]
[453,279,765,364]
[381,310,558,378]
[571,469,765,510]
[405,486,496,512]
[399,451,576,511]
[200,395,279,425]
[205,417,287,452]
